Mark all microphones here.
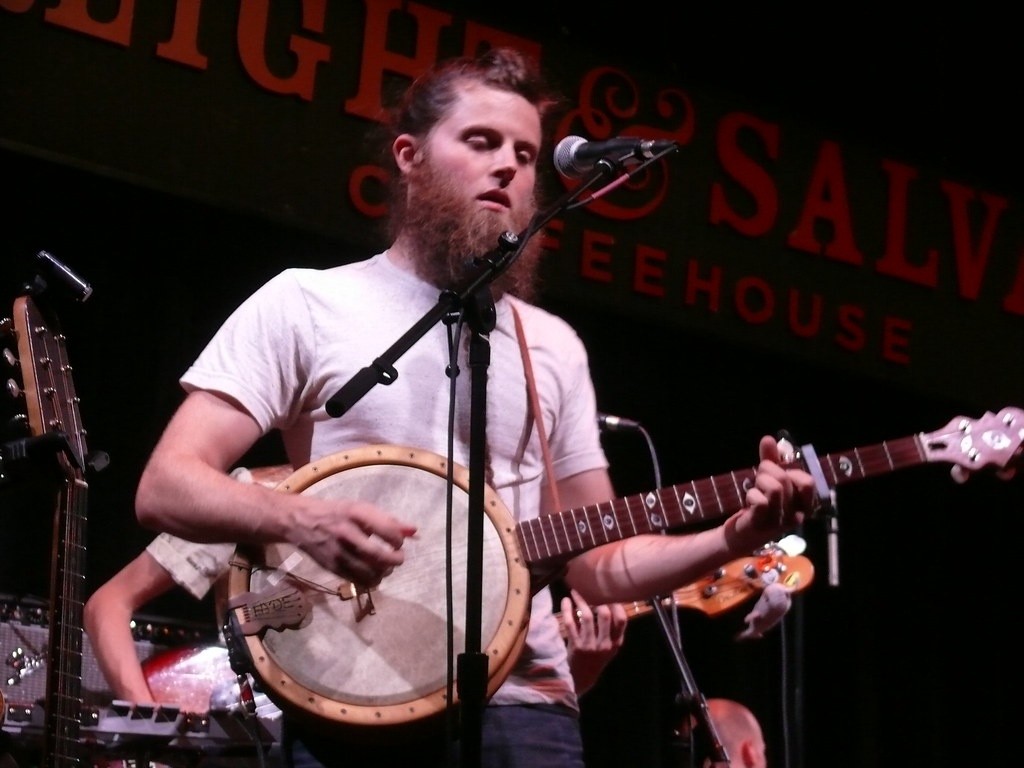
[825,485,840,589]
[554,136,678,179]
[597,412,642,433]
[7,655,47,685]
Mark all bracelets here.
[724,509,766,558]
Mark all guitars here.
[98,532,816,712]
[3,290,95,768]
[222,404,1024,731]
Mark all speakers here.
[0,593,218,709]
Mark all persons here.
[81,51,821,768]
